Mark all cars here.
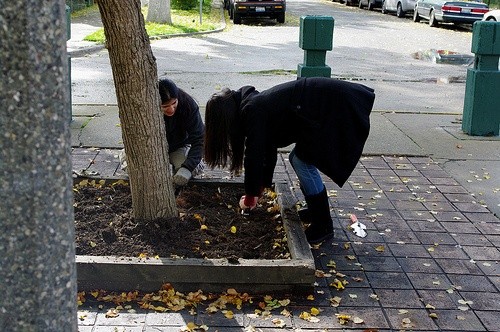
[223,0,286,24]
[331,0,500,27]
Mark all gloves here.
[173,167,192,186]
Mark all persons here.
[203,77,375,243]
[119,76,205,186]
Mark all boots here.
[304,185,334,245]
[298,184,310,223]
